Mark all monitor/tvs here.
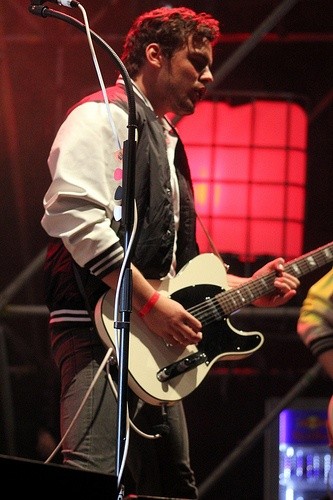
[263,398,333,500]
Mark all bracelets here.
[135,286,167,323]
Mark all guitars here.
[94,241,333,407]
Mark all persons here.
[44,7,298,500]
[288,259,333,473]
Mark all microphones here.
[31,0,80,9]
